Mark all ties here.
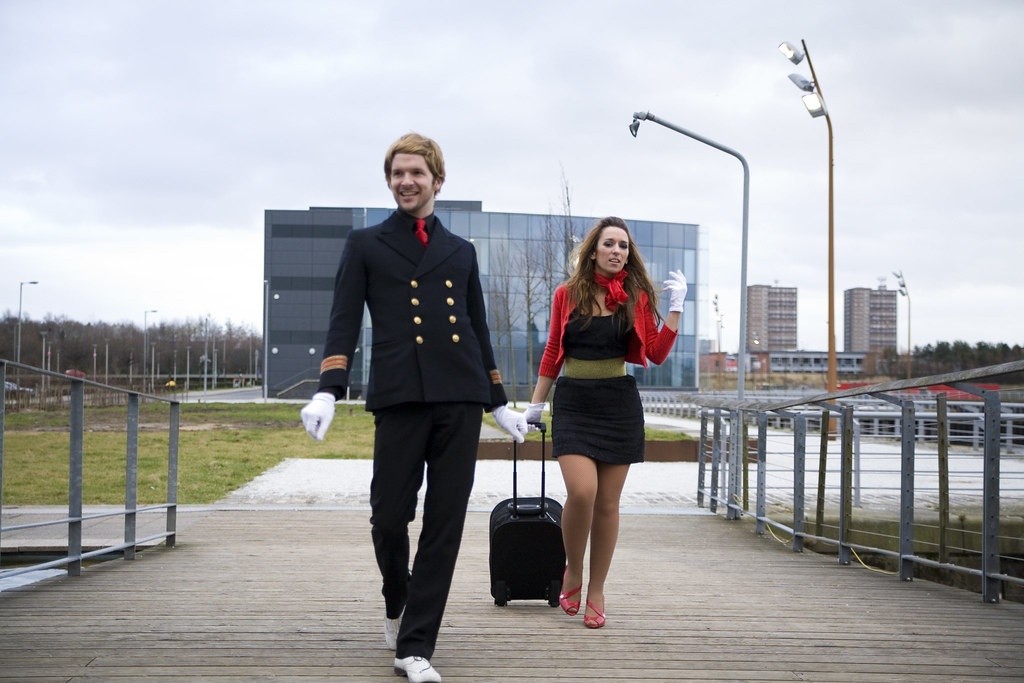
[413,217,429,246]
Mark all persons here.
[523,216,688,628]
[300,133,528,683]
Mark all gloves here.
[664,269,688,313]
[492,405,528,443]
[523,402,545,430]
[301,391,337,441]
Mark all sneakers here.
[394,656,442,683]
[384,605,407,650]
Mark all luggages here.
[489,422,567,608]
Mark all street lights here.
[12,278,218,404]
[776,37,838,443]
[893,268,912,379]
[629,111,750,403]
[711,294,727,388]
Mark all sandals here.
[559,564,582,616]
[583,592,606,628]
